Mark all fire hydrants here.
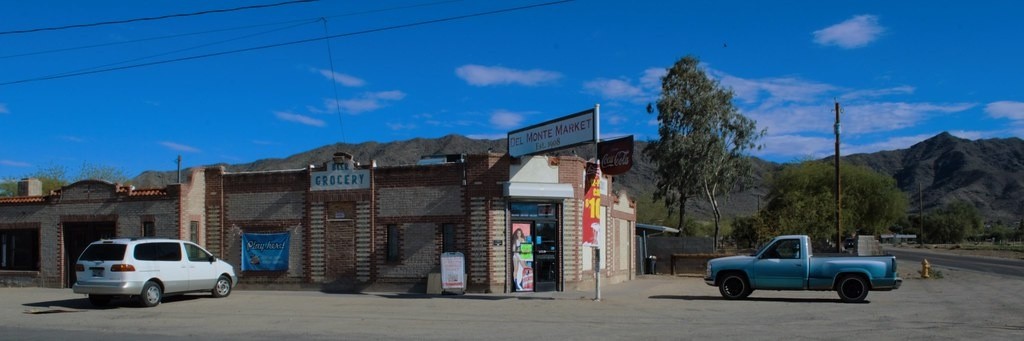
[921,258,931,278]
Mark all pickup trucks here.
[704,235,903,303]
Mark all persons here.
[512,228,527,290]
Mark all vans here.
[72,238,237,306]
